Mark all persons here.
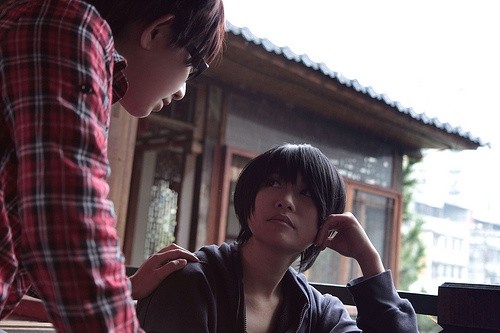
[134,142,420,333]
[0,0,224,333]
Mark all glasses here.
[181,37,210,83]
